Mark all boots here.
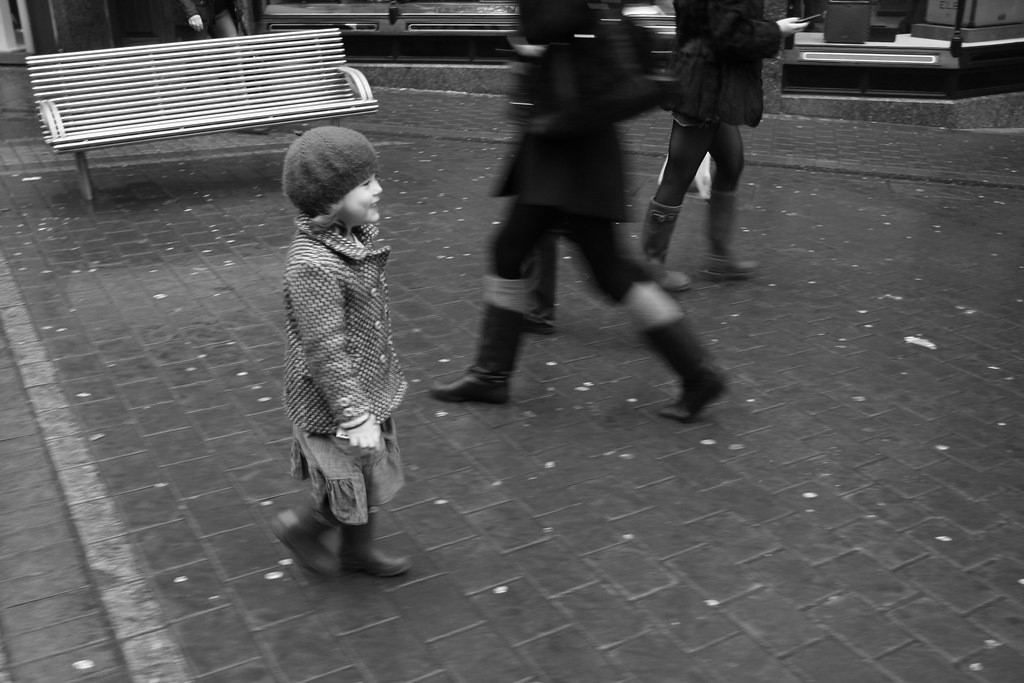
[432,302,557,405]
[337,507,410,578]
[699,189,757,279]
[638,196,693,291]
[270,497,340,573]
[638,317,727,421]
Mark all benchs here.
[25,25,380,201]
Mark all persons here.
[274,125,413,580]
[183,0,272,134]
[430,0,730,422]
[639,0,811,290]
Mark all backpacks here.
[596,19,656,120]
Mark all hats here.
[282,126,377,216]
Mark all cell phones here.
[795,14,822,23]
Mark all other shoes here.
[527,320,555,333]
